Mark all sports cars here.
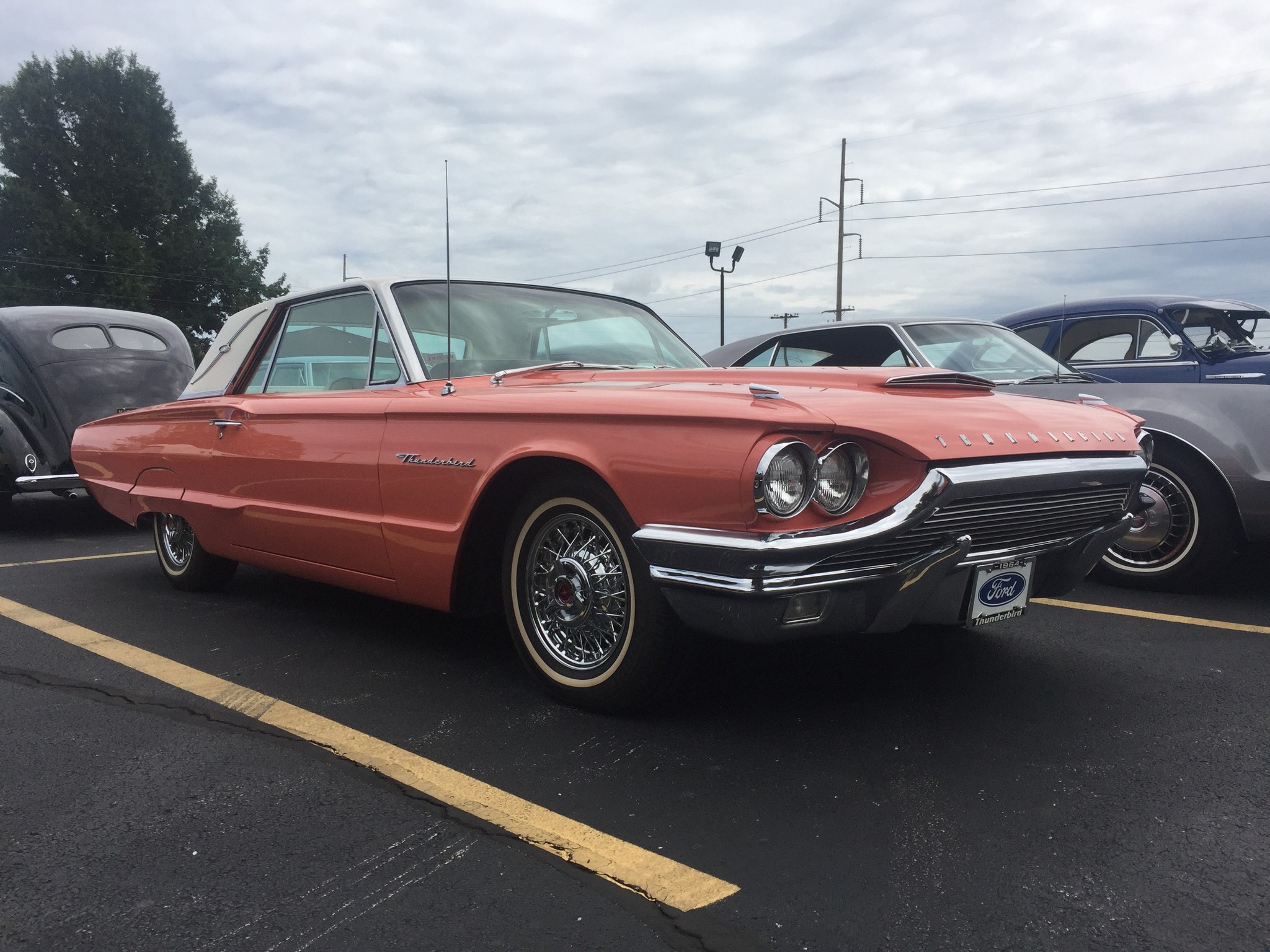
[71,272,1157,719]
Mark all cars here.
[936,294,1270,384]
[702,312,1270,598]
[243,356,432,395]
[0,305,196,531]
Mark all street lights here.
[705,240,745,348]
